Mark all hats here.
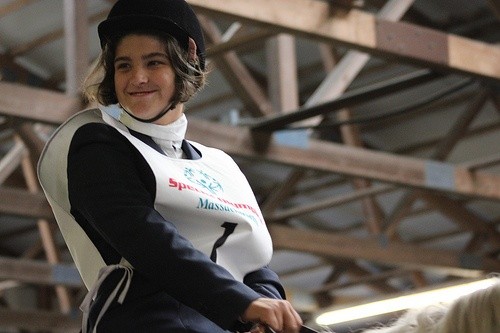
[96,0,206,79]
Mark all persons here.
[36,0,304,333]
